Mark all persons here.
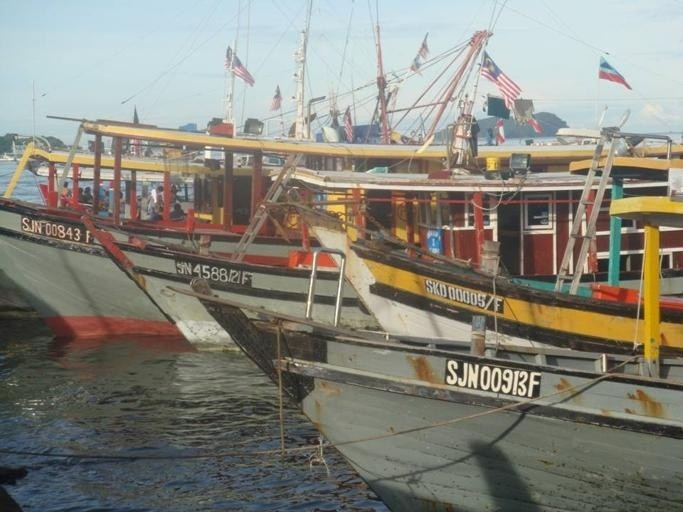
[58,180,186,221]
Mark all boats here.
[147,193,683,512]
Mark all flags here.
[224,45,281,112]
[598,53,632,90]
[342,105,352,144]
[479,50,543,145]
